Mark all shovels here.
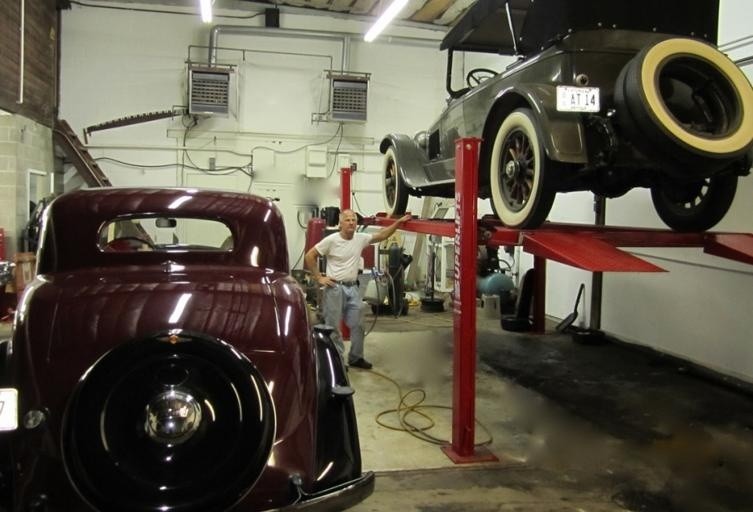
[556,284,586,338]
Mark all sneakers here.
[349,357,372,370]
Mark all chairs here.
[109,220,233,249]
[553,20,688,35]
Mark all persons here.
[302,208,412,372]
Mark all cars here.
[0,185,378,510]
[376,0,752,235]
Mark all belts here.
[330,279,358,286]
